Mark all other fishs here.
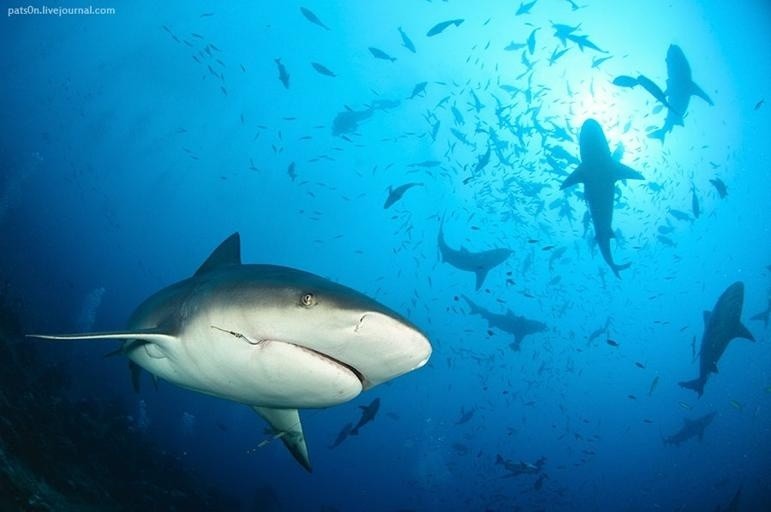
[161,0,771,512]
